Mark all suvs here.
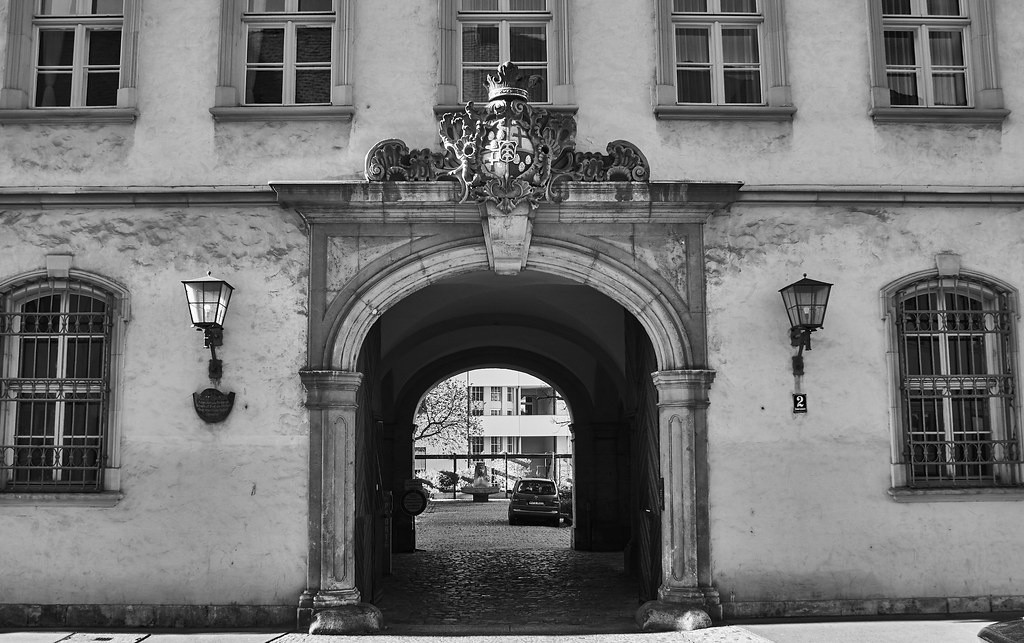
[508,476,561,524]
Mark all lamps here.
[181,270,236,380]
[778,274,834,377]
